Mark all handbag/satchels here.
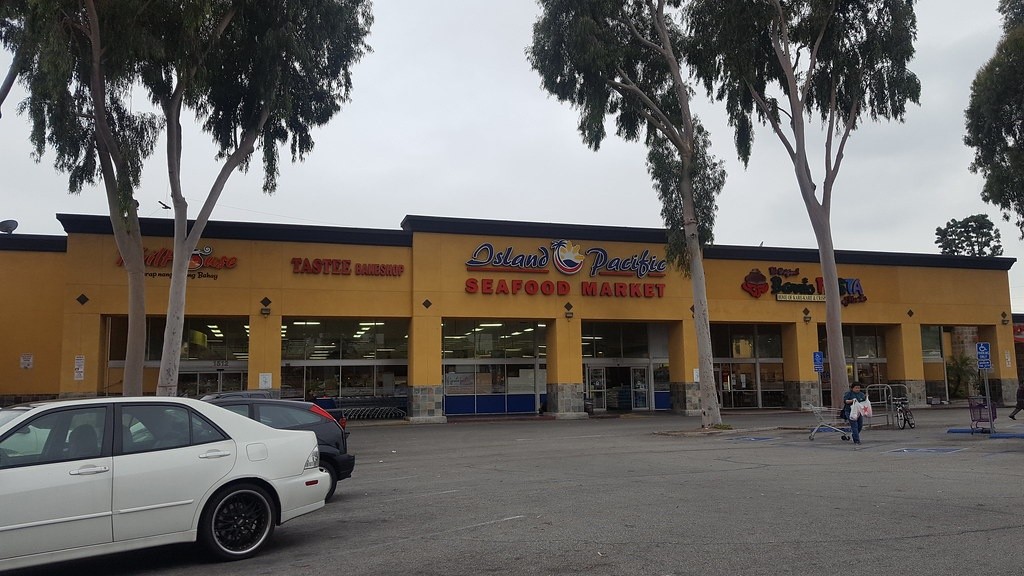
[849,396,873,421]
[840,410,847,420]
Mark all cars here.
[0,396,337,574]
[1,406,77,459]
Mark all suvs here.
[137,392,355,499]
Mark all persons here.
[843,382,866,444]
[306,389,315,402]
[1009,380,1024,420]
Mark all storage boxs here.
[927,397,941,405]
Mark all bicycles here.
[889,394,918,428]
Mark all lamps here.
[1003,319,1009,325]
[804,316,811,322]
[565,312,573,318]
[261,307,270,314]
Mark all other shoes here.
[851,433,854,442]
[1009,415,1015,419]
[854,440,861,445]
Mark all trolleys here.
[966,394,998,434]
[806,402,851,441]
